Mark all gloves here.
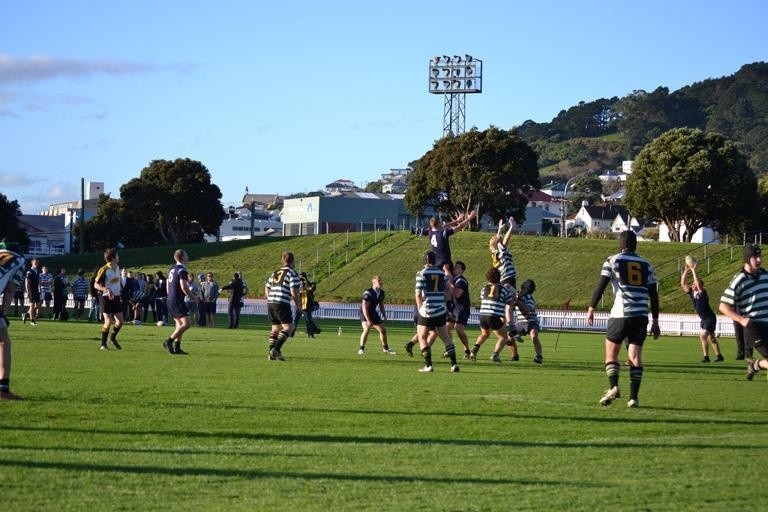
[650,317,660,339]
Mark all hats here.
[742,243,761,263]
[619,230,637,251]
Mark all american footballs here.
[685,256,696,267]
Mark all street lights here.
[560,169,594,238]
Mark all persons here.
[405,210,542,373]
[718,244,768,380]
[586,231,660,406]
[289,272,322,338]
[729,267,753,360]
[94,247,123,351]
[680,263,725,363]
[357,275,396,355]
[0,236,24,400]
[264,251,302,361]
[13,267,248,329]
[163,249,200,354]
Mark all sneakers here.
[100,345,107,351]
[702,357,755,380]
[160,339,175,356]
[356,335,544,374]
[22,316,239,330]
[172,348,187,355]
[108,336,121,351]
[1,389,21,399]
[599,385,620,406]
[272,349,284,361]
[627,398,639,407]
